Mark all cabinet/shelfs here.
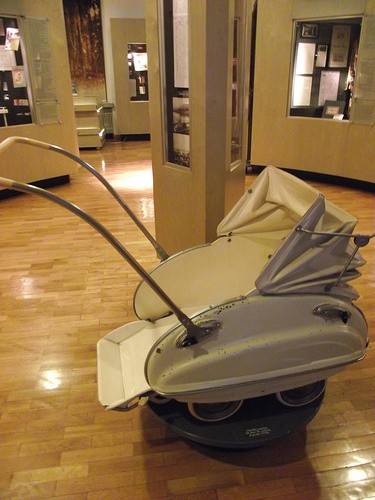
[71,95,107,151]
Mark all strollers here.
[0,135,374,452]
